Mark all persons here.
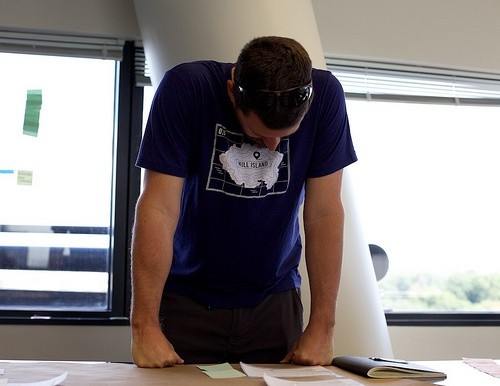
[129,36,359,370]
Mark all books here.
[330,356,447,383]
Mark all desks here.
[0,360,499,386]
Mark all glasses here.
[234,78,314,110]
